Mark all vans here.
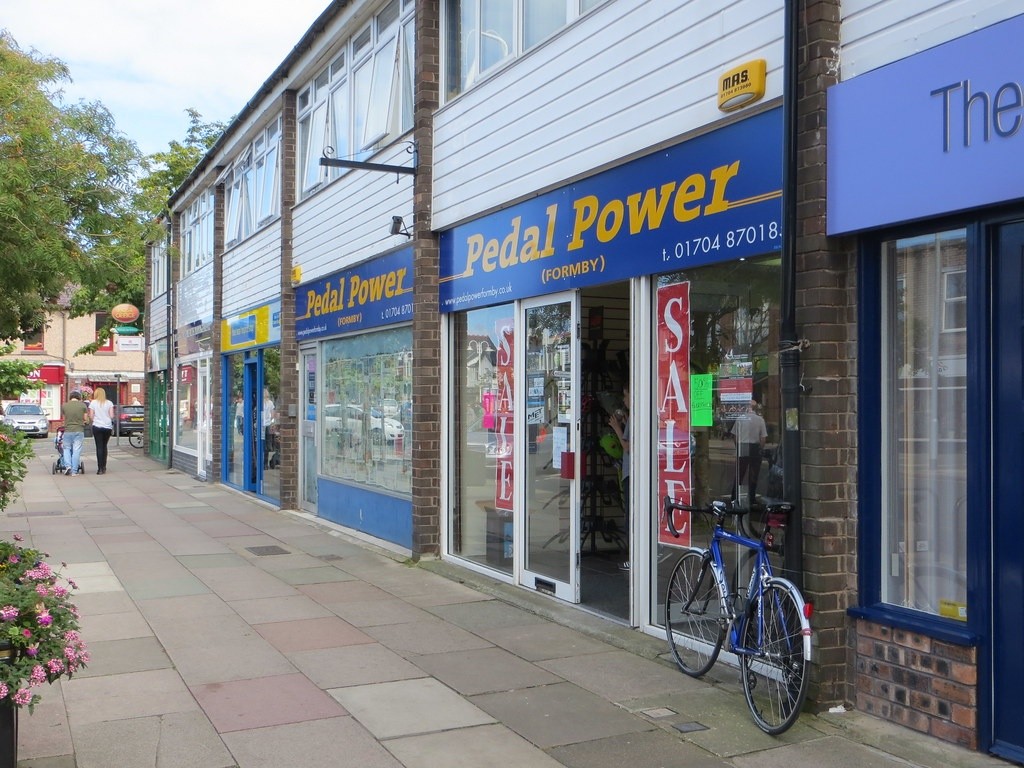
[377,399,399,419]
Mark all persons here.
[729,399,767,503]
[263,388,276,468]
[609,381,629,571]
[61,391,88,476]
[236,391,243,436]
[89,387,114,474]
[132,397,141,406]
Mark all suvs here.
[112,404,145,436]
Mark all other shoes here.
[72,472,80,477]
[97,467,106,475]
[264,465,268,470]
[64,467,71,475]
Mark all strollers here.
[51,427,85,475]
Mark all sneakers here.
[657,549,673,565]
[618,559,630,570]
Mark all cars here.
[0,402,51,438]
[158,405,184,438]
[324,403,405,448]
[653,413,698,469]
[356,404,384,419]
[758,440,784,499]
[402,400,412,419]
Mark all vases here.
[0,638,26,768]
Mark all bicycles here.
[129,427,143,449]
[663,492,813,737]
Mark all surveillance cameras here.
[390,216,403,235]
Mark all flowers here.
[0,531,95,704]
